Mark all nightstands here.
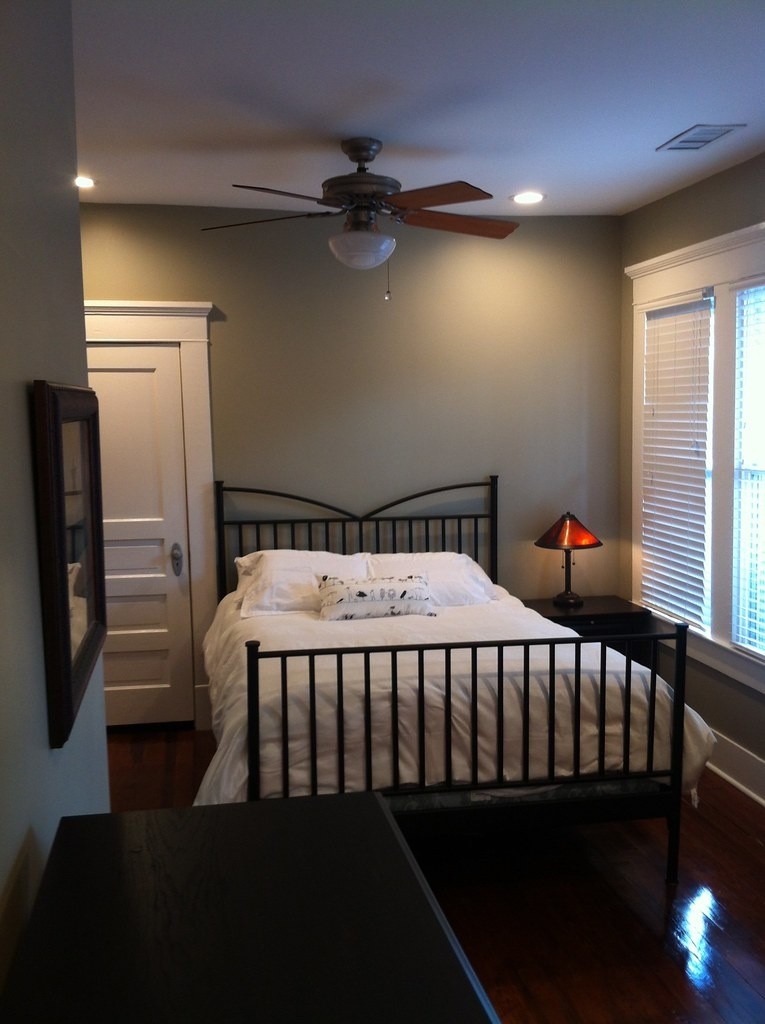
[521,595,653,667]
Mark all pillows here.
[368,551,500,606]
[235,550,373,619]
[316,571,437,622]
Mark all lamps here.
[534,511,604,608]
[329,210,397,302]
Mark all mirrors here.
[30,379,108,749]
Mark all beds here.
[194,476,718,893]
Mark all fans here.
[201,137,520,240]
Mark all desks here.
[2,793,500,1024]
